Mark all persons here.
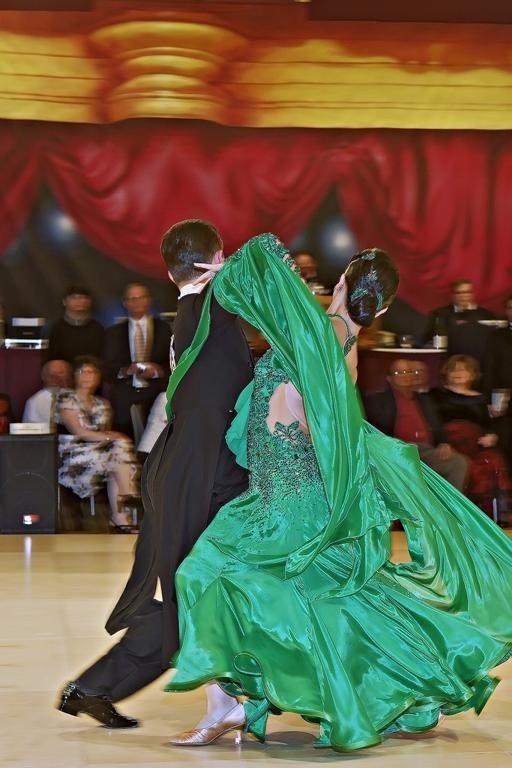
[355,276,510,535]
[28,282,175,534]
[55,219,402,748]
[295,253,317,281]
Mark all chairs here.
[56,463,139,526]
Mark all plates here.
[478,320,507,326]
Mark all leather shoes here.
[56,681,137,728]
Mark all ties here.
[134,321,146,385]
[49,391,56,433]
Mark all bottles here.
[432,313,448,349]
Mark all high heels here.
[107,517,140,534]
[117,493,143,513]
[169,702,247,746]
